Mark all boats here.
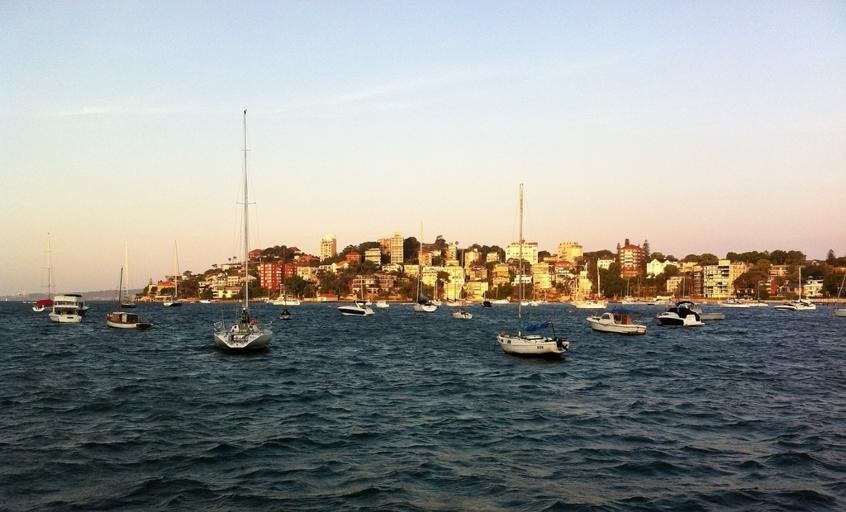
[452,311,473,320]
[720,297,769,308]
[773,299,816,311]
[495,333,570,356]
[413,301,442,312]
[106,311,151,330]
[834,307,846,317]
[120,301,137,308]
[48,295,89,323]
[163,296,182,307]
[32,307,46,312]
[21,301,30,303]
[445,298,474,307]
[586,313,647,336]
[337,302,389,317]
[655,301,725,327]
[280,314,292,320]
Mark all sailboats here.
[213,108,273,351]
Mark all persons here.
[241,308,249,322]
[281,308,291,315]
